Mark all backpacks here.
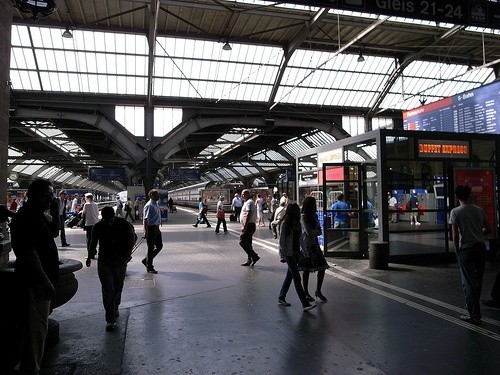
[202,202,208,214]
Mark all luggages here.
[230,214,235,222]
[65,216,80,228]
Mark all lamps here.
[11,0,58,23]
[357,54,366,62]
[223,42,232,50]
[62,29,73,39]
[468,65,473,71]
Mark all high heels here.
[305,295,315,301]
[315,290,327,301]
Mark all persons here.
[193,197,211,228]
[9,199,17,213]
[167,198,174,213]
[55,190,86,247]
[447,185,492,323]
[408,193,421,225]
[10,180,62,375]
[276,197,330,311]
[116,197,142,223]
[142,189,163,274]
[80,192,99,259]
[481,205,500,309]
[388,195,397,223]
[238,189,261,266]
[231,193,289,240]
[351,200,376,228]
[216,195,229,234]
[331,194,355,239]
[86,206,138,330]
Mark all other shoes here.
[303,304,317,312]
[216,232,221,235]
[481,299,500,309]
[241,256,260,267]
[277,298,291,306]
[206,225,211,228]
[256,223,259,226]
[62,242,70,247]
[193,224,198,228]
[106,310,120,332]
[142,258,158,274]
[415,222,421,226]
[411,222,414,225]
[459,311,481,322]
[225,231,229,234]
[261,224,265,227]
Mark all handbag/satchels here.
[217,208,225,219]
[124,205,132,211]
[77,213,86,227]
[200,217,205,224]
